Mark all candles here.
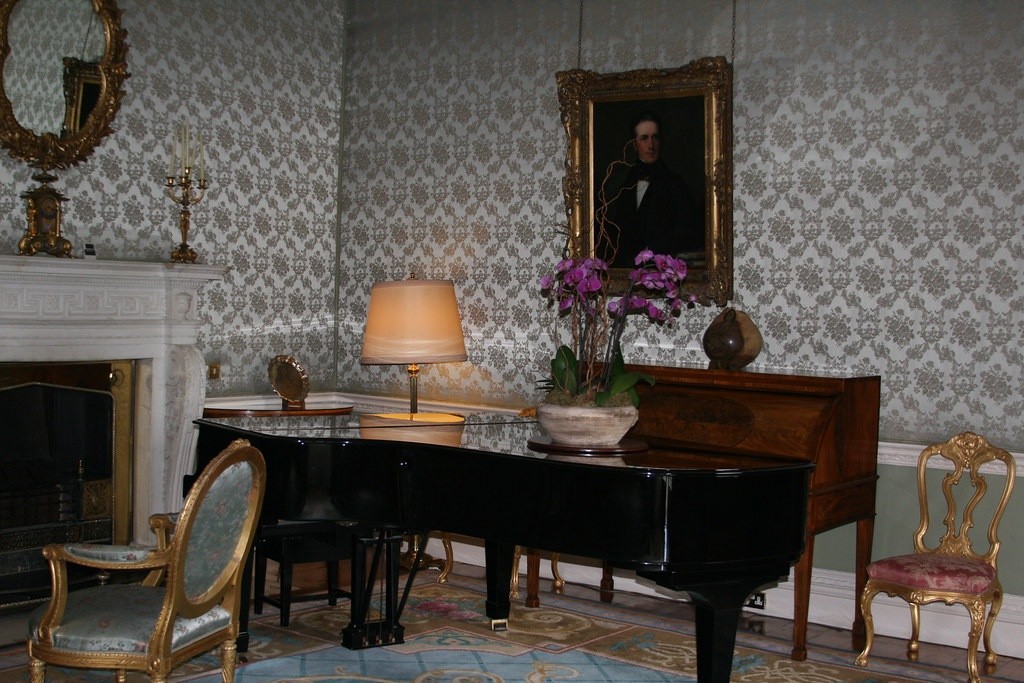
[167,118,209,180]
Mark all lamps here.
[359,406,466,448]
[356,271,468,414]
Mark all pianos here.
[182,409,818,683]
[512,354,885,664]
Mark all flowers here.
[538,138,698,408]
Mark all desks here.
[176,409,817,682]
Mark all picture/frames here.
[59,56,102,140]
[556,55,737,306]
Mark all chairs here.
[854,430,1016,683]
[28,436,269,683]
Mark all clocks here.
[20,171,73,259]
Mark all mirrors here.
[0,0,134,168]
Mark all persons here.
[607,112,699,269]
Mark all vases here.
[536,400,640,444]
